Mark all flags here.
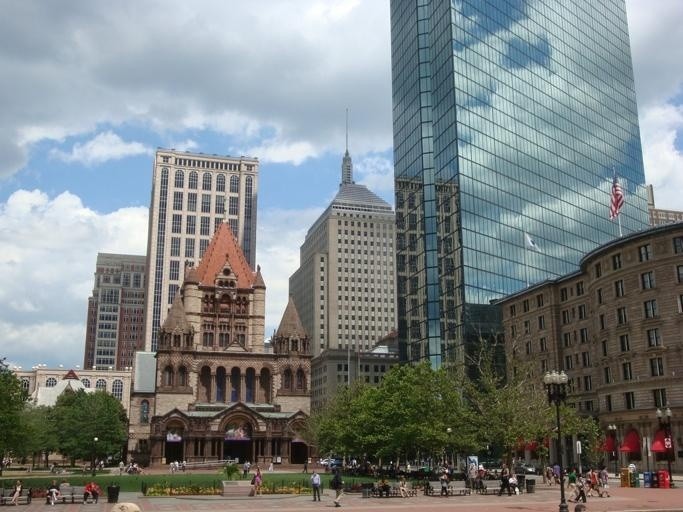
[525,233,540,251]
[609,173,624,220]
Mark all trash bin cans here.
[362,482,374,498]
[107,486,120,503]
[526,479,535,493]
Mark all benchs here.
[373,479,514,497]
[0,488,31,505]
[46,486,101,505]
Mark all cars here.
[514,463,536,474]
[317,458,335,467]
[478,461,505,480]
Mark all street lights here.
[543,369,570,512]
[655,406,675,488]
[91,433,100,468]
[607,421,621,478]
